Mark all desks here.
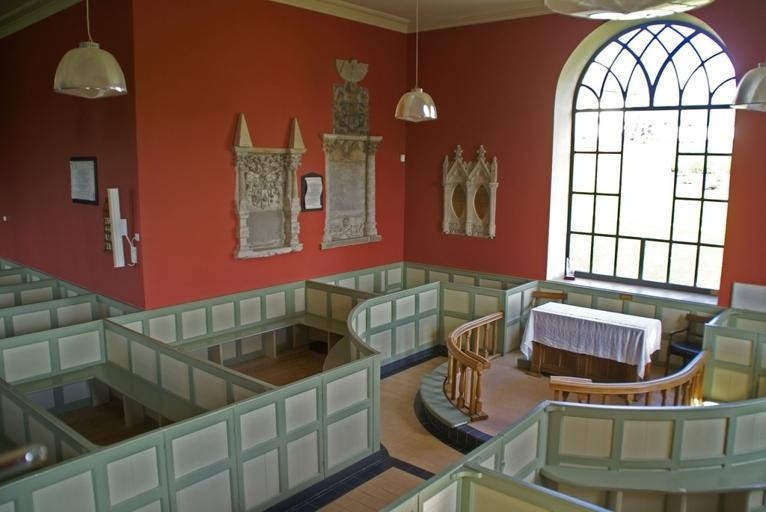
[527,300,663,402]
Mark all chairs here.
[665,313,714,377]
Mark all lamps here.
[53,0,129,100]
[393,0,439,121]
[728,62,766,113]
